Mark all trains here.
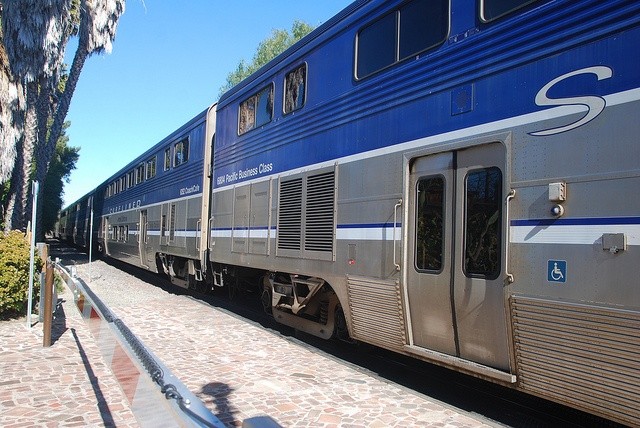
[53,0,640,428]
